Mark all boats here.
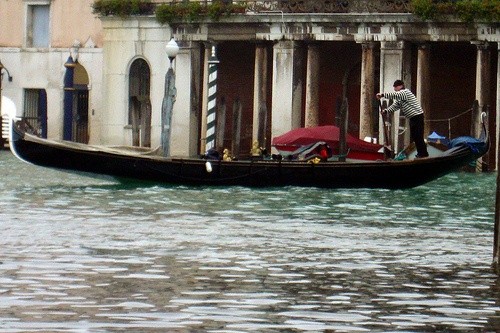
[8,106,491,192]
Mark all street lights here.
[161,36,180,152]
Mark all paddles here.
[378,99,389,146]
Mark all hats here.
[393,80,404,86]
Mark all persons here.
[377,80,430,160]
